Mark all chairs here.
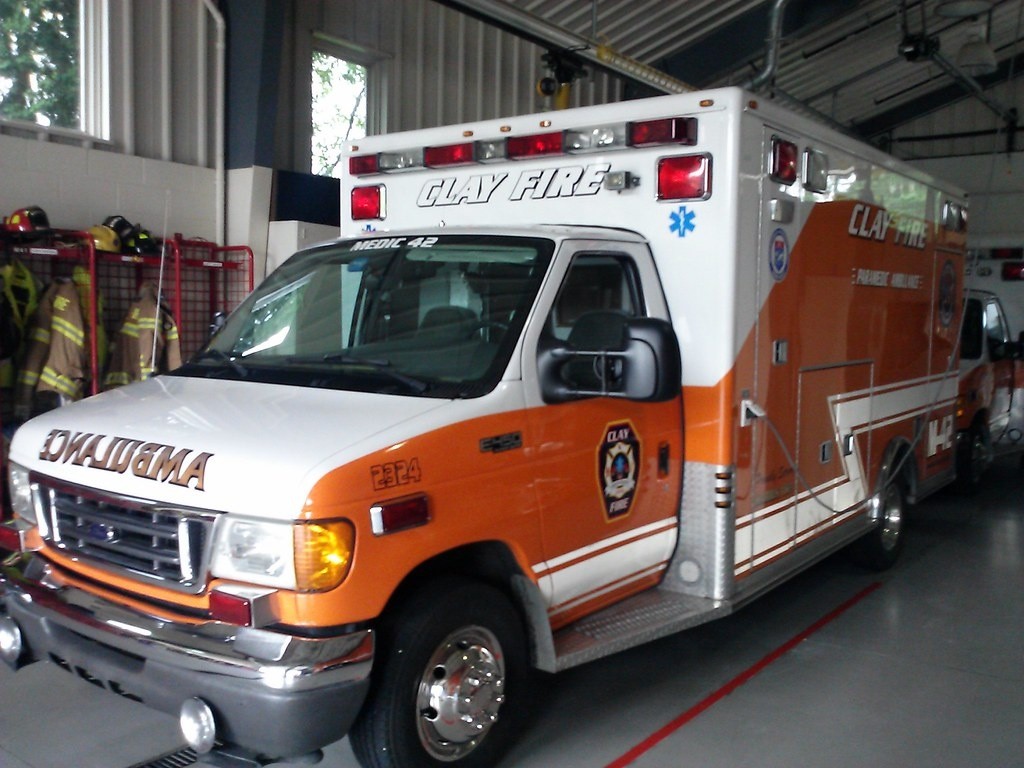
[413,307,479,366]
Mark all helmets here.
[7,205,50,235]
[81,216,159,257]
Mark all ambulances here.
[957,258,1024,462]
[0,86,975,768]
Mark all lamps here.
[933,0,996,18]
[955,21,997,77]
